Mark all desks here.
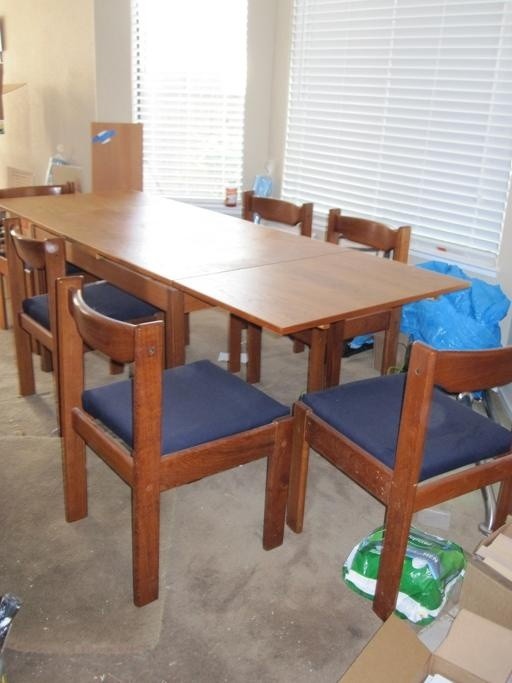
[0,189,470,394]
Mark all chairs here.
[55,276,297,606]
[1,216,169,396]
[287,341,511,623]
[289,209,413,391]
[185,191,314,383]
[1,592,22,650]
[1,182,74,354]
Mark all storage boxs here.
[335,513,512,683]
